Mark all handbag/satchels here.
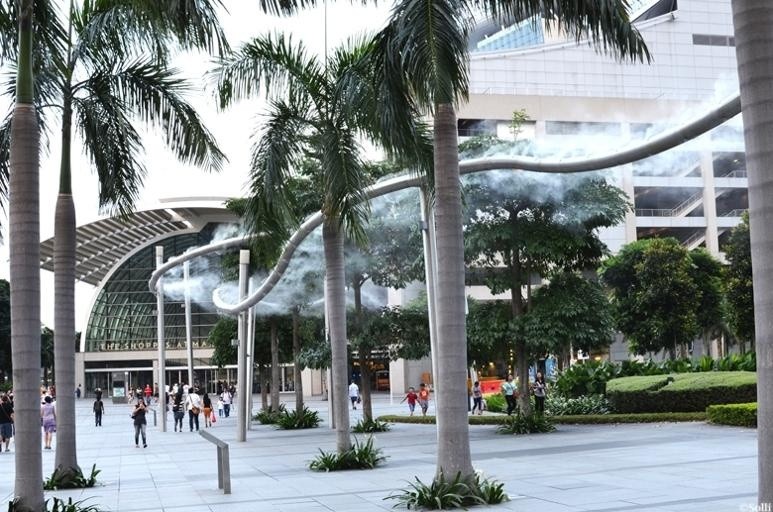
[192,406,199,415]
[173,406,178,412]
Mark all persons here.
[470,380,484,415]
[348,379,359,410]
[127,383,152,406]
[399,386,421,415]
[131,396,148,448]
[152,380,235,432]
[92,395,104,426]
[0,377,56,454]
[531,371,545,414]
[76,383,81,399]
[418,382,429,415]
[501,375,518,415]
